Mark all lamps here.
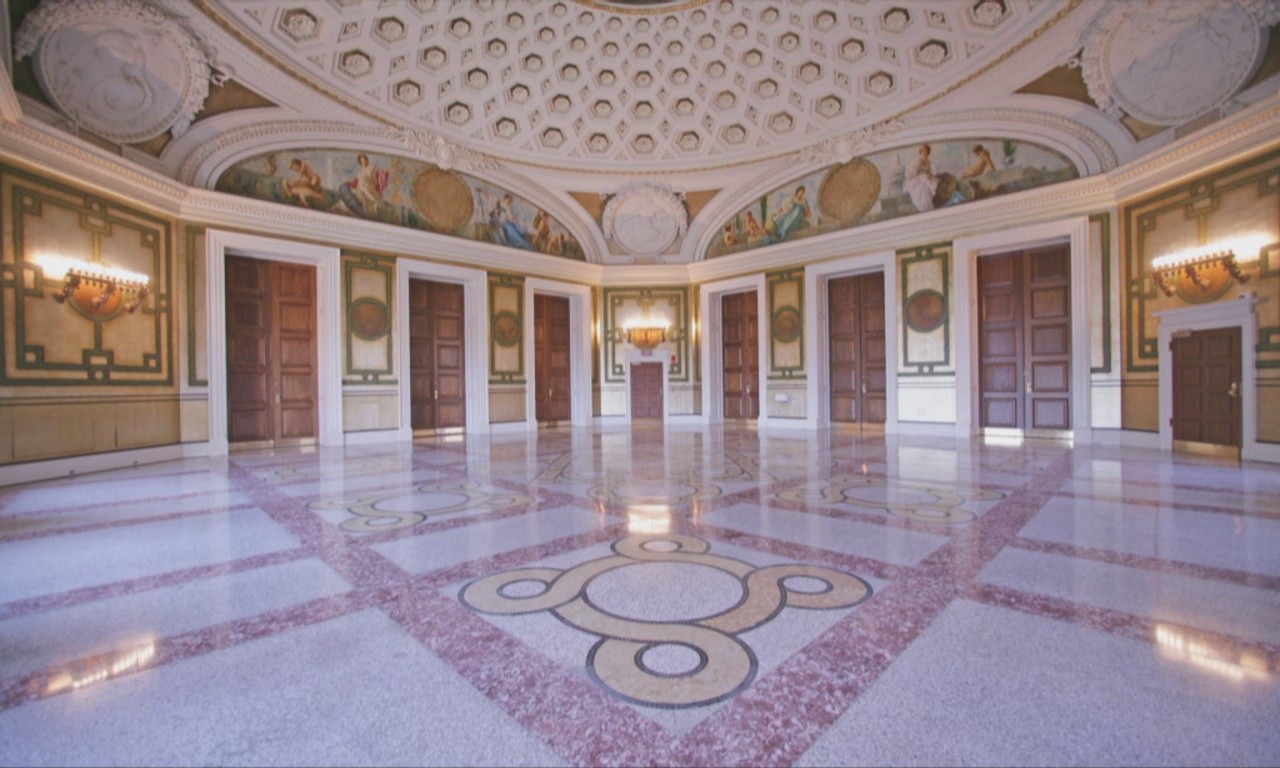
[1153,250,1250,304]
[627,327,665,349]
[54,267,151,312]
